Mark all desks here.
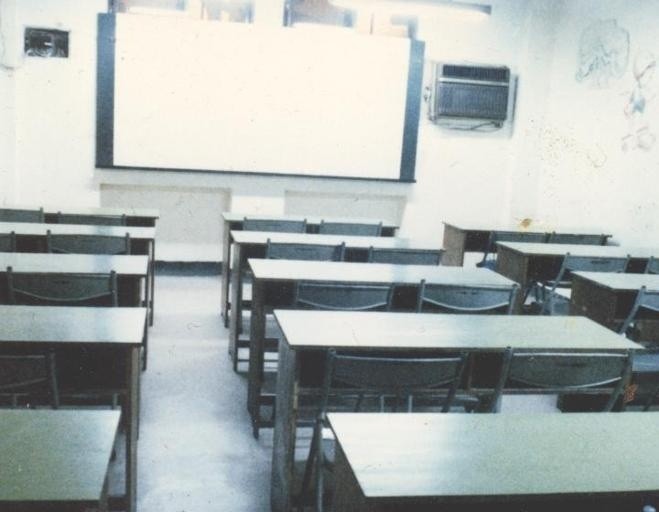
[0,204,162,512]
[216,213,659,512]
